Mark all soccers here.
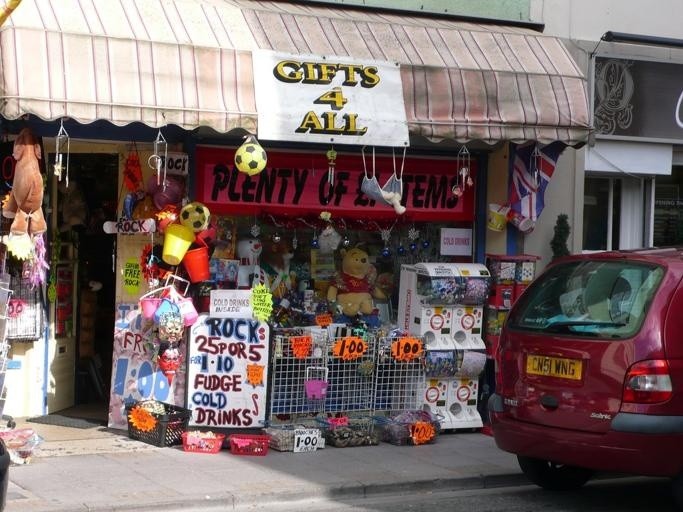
[181,202,210,231]
[235,143,267,175]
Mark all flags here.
[510,141,567,223]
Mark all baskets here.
[316,415,390,448]
[125,400,192,447]
[228,434,271,456]
[181,432,225,454]
[258,418,330,452]
[377,414,445,446]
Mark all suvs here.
[487,247,683,492]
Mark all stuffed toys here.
[380,190,405,214]
[237,238,270,291]
[271,242,294,273]
[57,179,86,234]
[1,126,47,237]
[326,248,387,316]
